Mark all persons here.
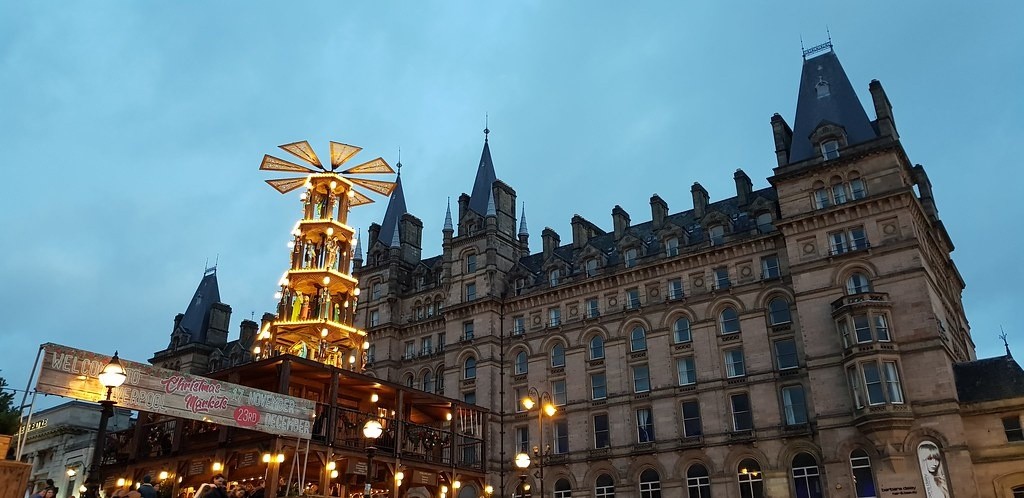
[179,474,265,498]
[278,477,318,496]
[32,479,58,498]
[302,192,336,216]
[290,236,339,268]
[283,286,358,320]
[161,433,172,456]
[919,444,950,498]
[110,474,162,498]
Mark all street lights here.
[523,387,556,498]
[363,420,382,498]
[83,350,128,498]
